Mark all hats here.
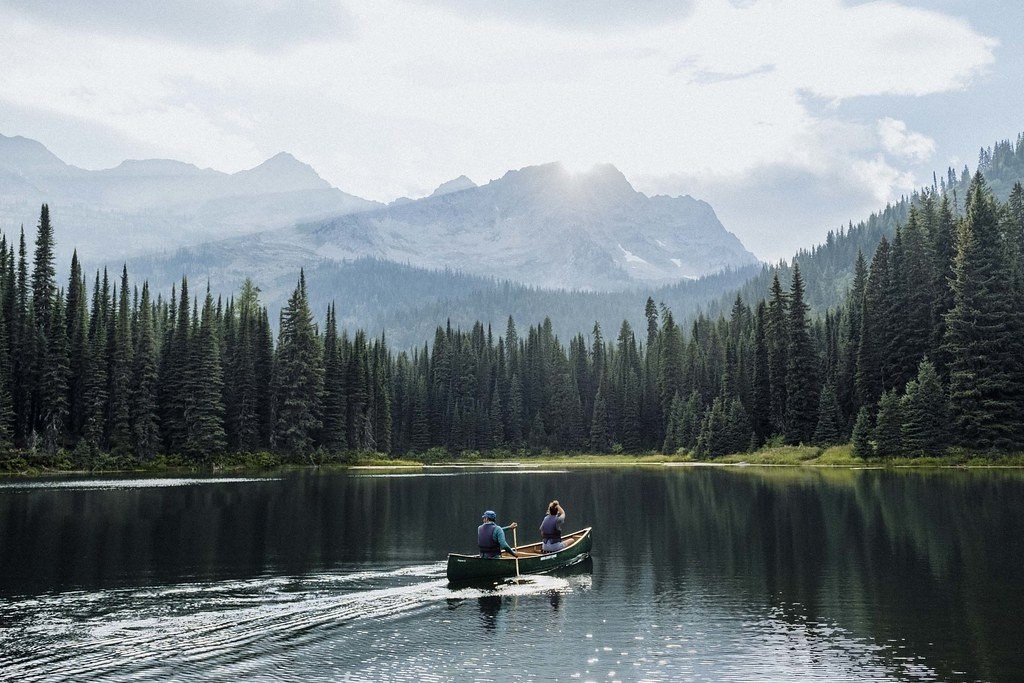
[481,510,496,519]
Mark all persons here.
[478,511,518,558]
[539,500,574,551]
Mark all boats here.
[445,526,592,582]
